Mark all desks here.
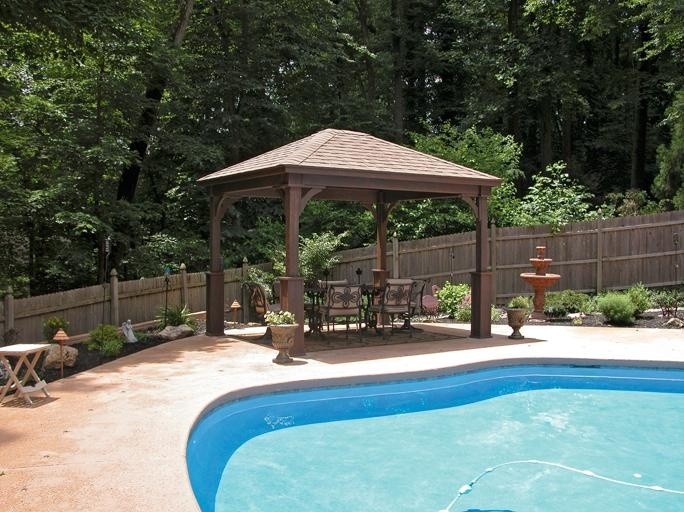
[0,342,52,405]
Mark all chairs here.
[241,276,430,344]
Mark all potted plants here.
[502,294,530,339]
[263,309,299,364]
